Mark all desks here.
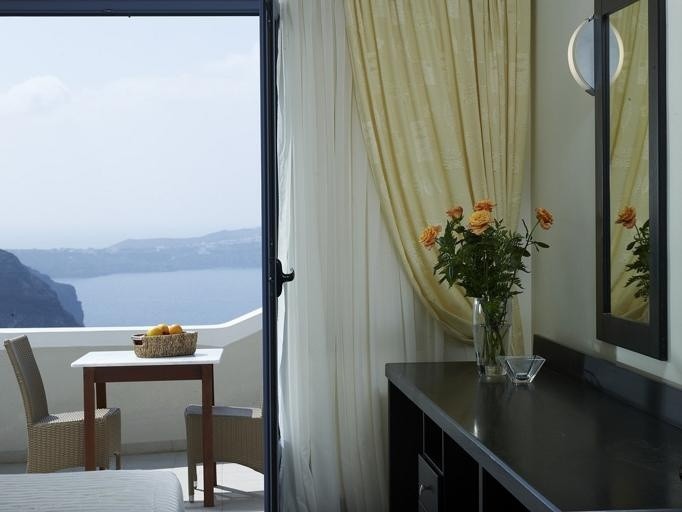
[71,348,226,507]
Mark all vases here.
[469,298,548,386]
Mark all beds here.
[1,469,185,512]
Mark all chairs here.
[3,333,122,474]
[184,403,265,503]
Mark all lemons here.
[146,324,182,336]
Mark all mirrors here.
[595,0,668,363]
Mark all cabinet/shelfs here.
[385,356,681,512]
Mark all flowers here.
[612,205,651,307]
[418,198,553,367]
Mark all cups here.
[485,322,546,386]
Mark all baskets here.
[131,331,199,359]
